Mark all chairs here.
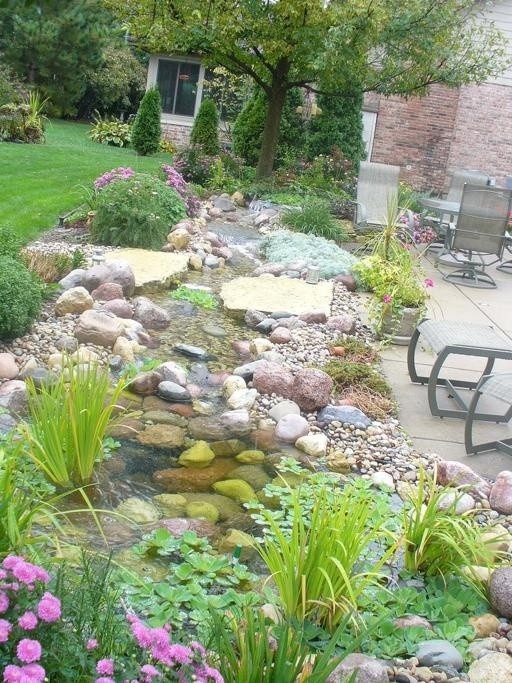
[439,172,495,252]
[497,228,512,270]
[352,161,413,243]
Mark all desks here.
[417,198,504,267]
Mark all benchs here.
[409,317,512,422]
[465,374,512,457]
